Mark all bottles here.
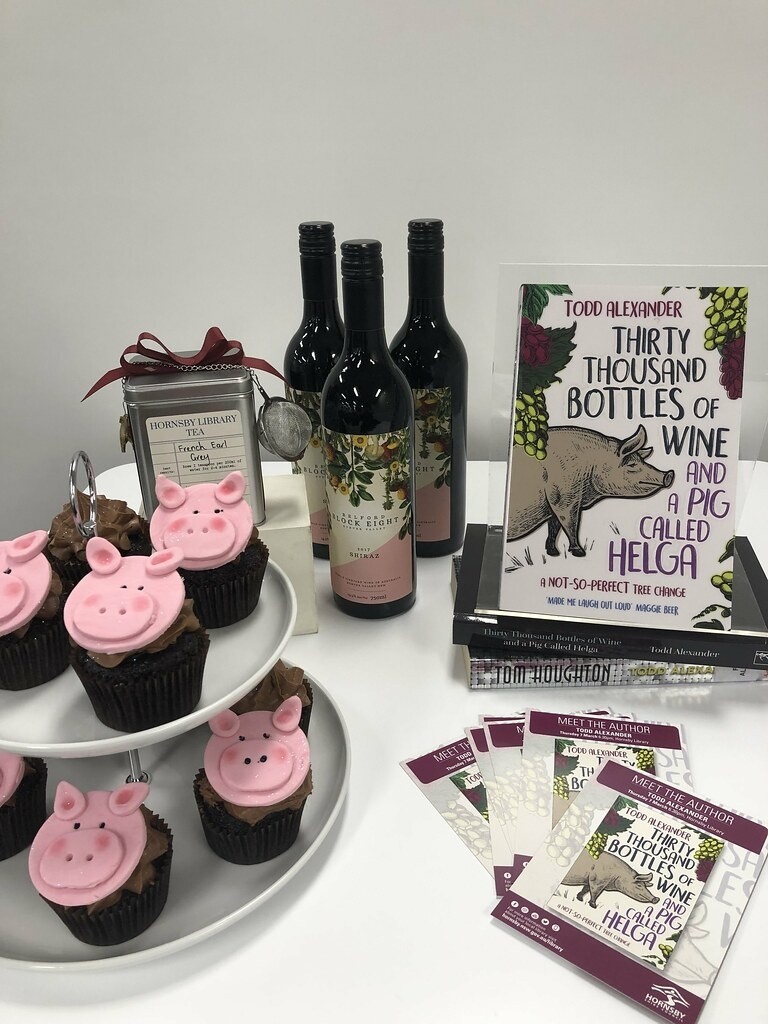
[284,221,346,561]
[321,238,418,620]
[388,218,469,558]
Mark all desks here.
[0,457,768,1024]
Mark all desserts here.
[0,469,270,735]
[0,750,176,947]
[194,658,315,863]
